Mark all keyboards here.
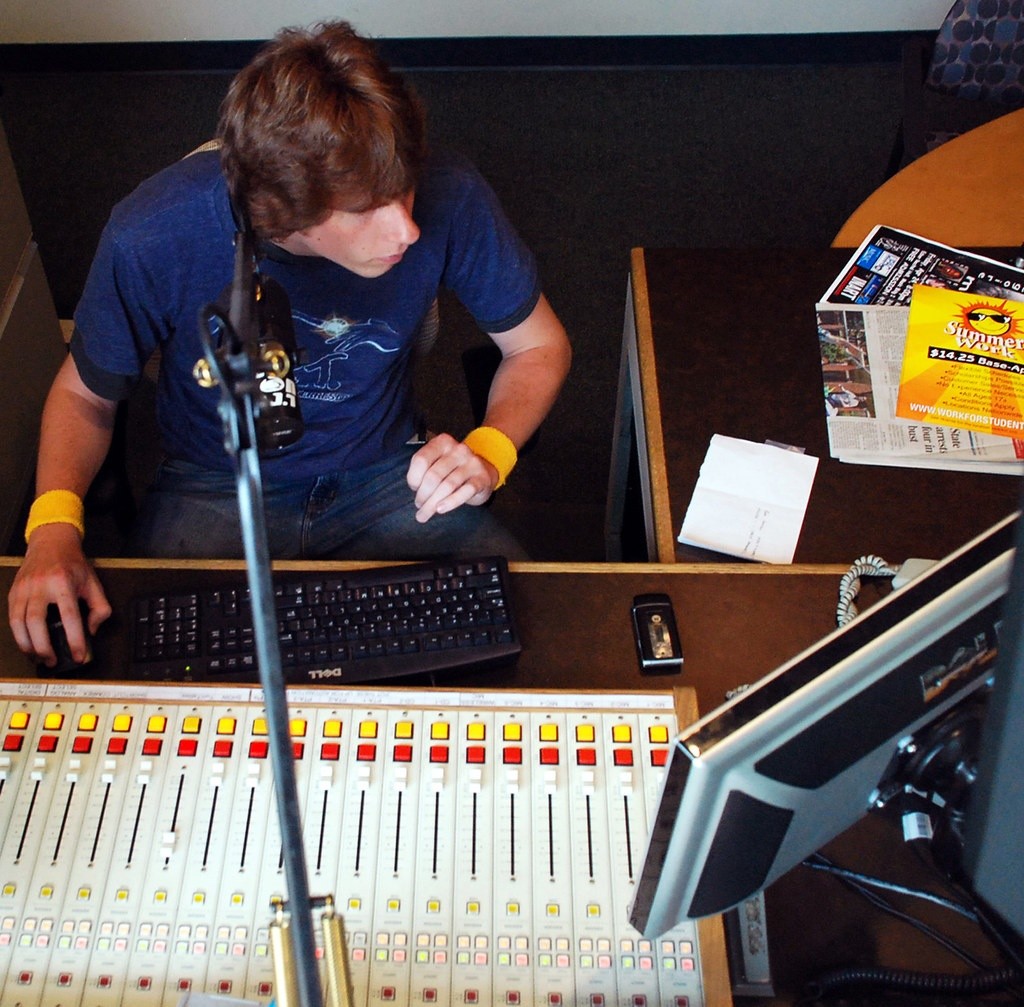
[127,553,525,684]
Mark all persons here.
[936,261,963,280]
[826,382,867,410]
[924,276,951,290]
[8,20,569,668]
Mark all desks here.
[0,557,1024,1007]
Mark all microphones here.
[243,276,303,458]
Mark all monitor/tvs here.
[631,509,1024,939]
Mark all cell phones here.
[632,592,684,675]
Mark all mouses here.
[28,594,100,681]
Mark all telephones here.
[837,553,941,629]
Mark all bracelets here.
[462,427,517,490]
[24,490,84,541]
